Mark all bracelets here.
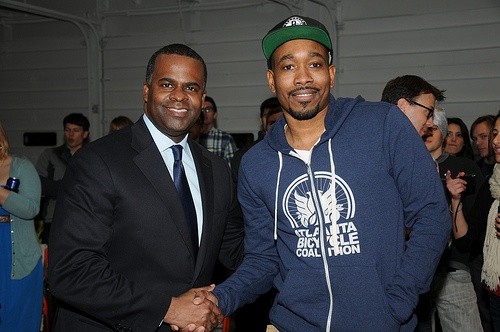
[449,203,463,212]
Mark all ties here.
[169,144,198,266]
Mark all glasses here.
[201,106,215,112]
[410,100,434,119]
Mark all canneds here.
[5,176,20,192]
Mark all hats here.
[262,15,332,60]
[432,109,449,140]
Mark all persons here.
[443,118,474,176]
[446,111,500,332]
[256,96,286,147]
[381,74,445,140]
[265,109,285,132]
[32,112,89,245]
[467,115,497,251]
[0,127,46,332]
[412,106,485,332]
[41,43,278,331]
[173,14,453,332]
[109,116,131,132]
[200,95,238,175]
